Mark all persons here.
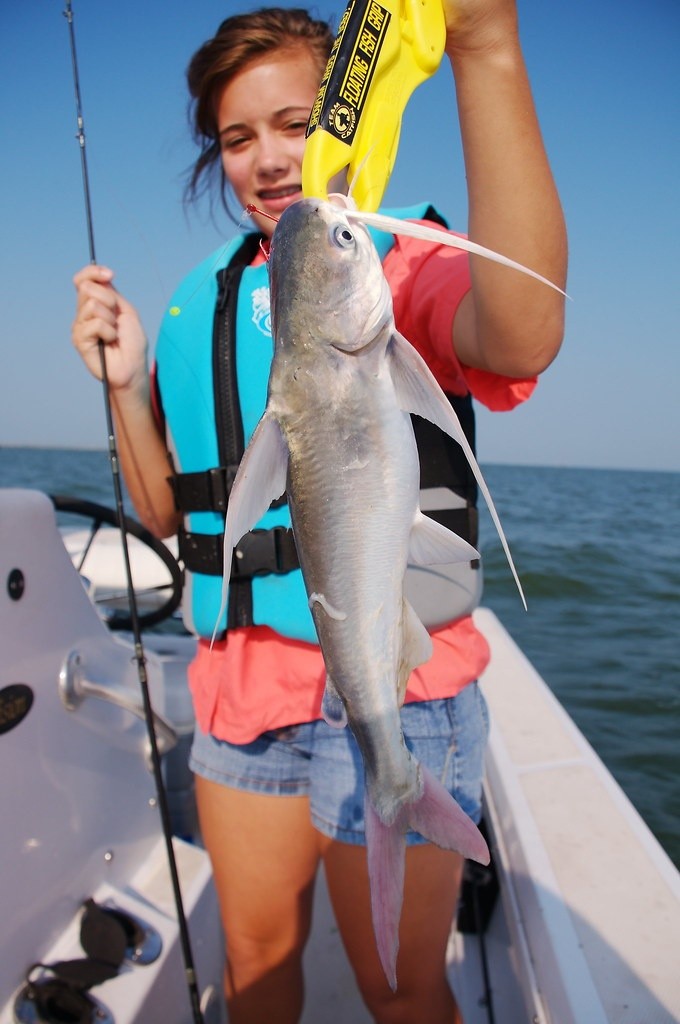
[69,1,567,1024]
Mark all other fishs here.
[205,193,571,993]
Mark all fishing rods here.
[62,0,207,1024]
